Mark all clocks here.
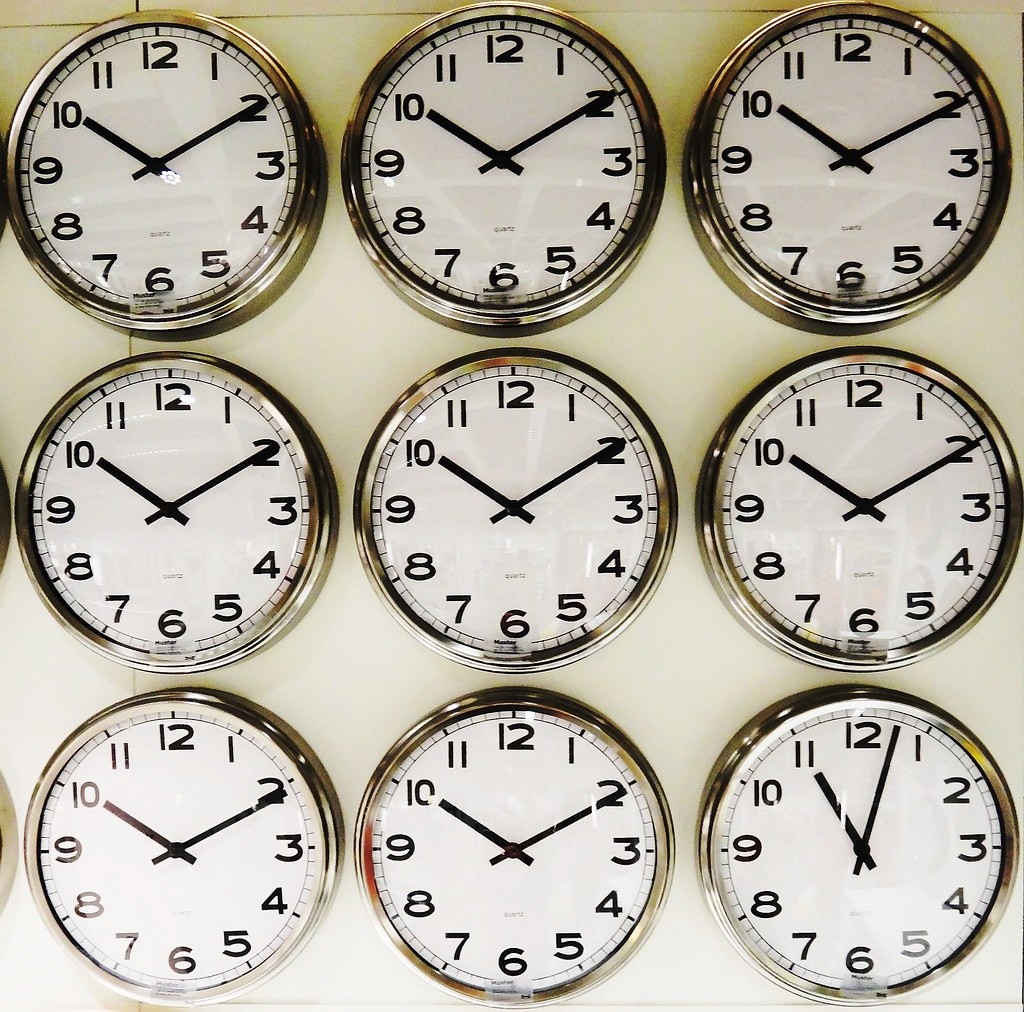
[25,689,345,1009]
[351,346,679,677]
[4,10,326,341]
[352,688,675,1009]
[14,349,339,674]
[339,2,669,338]
[694,685,1020,1006]
[678,0,1014,338]
[694,346,1024,675]
